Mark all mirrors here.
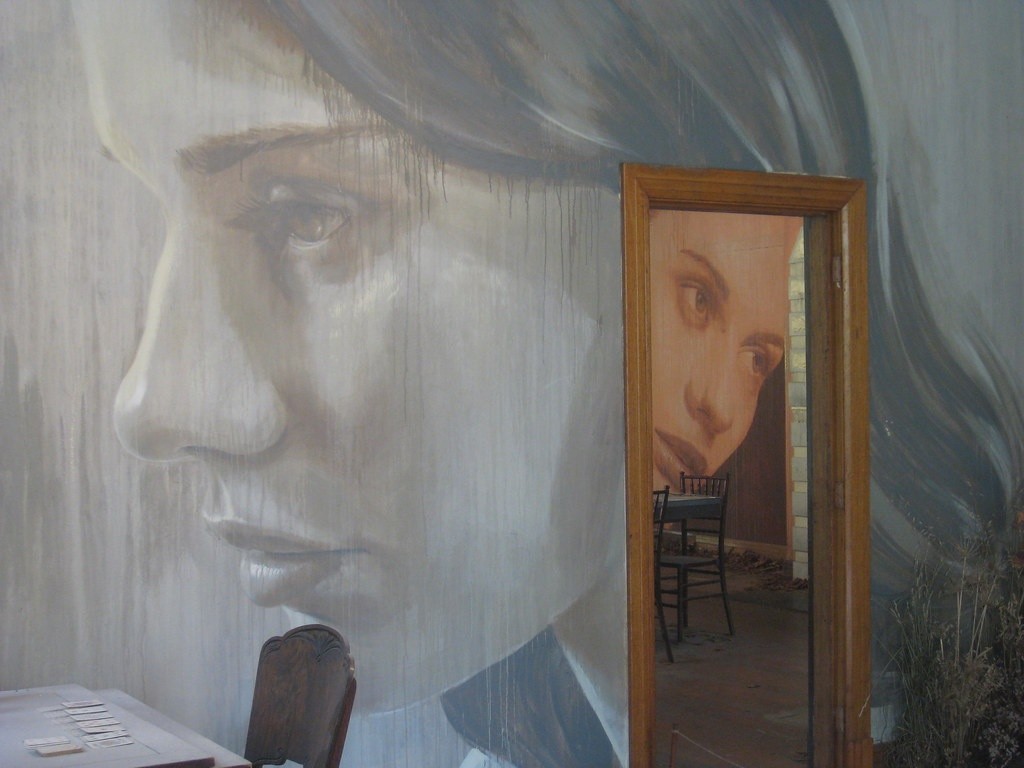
[622,160,873,768]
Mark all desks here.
[653,492,722,523]
[1,682,254,768]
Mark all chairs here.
[653,471,734,641]
[243,623,357,768]
[653,485,674,663]
